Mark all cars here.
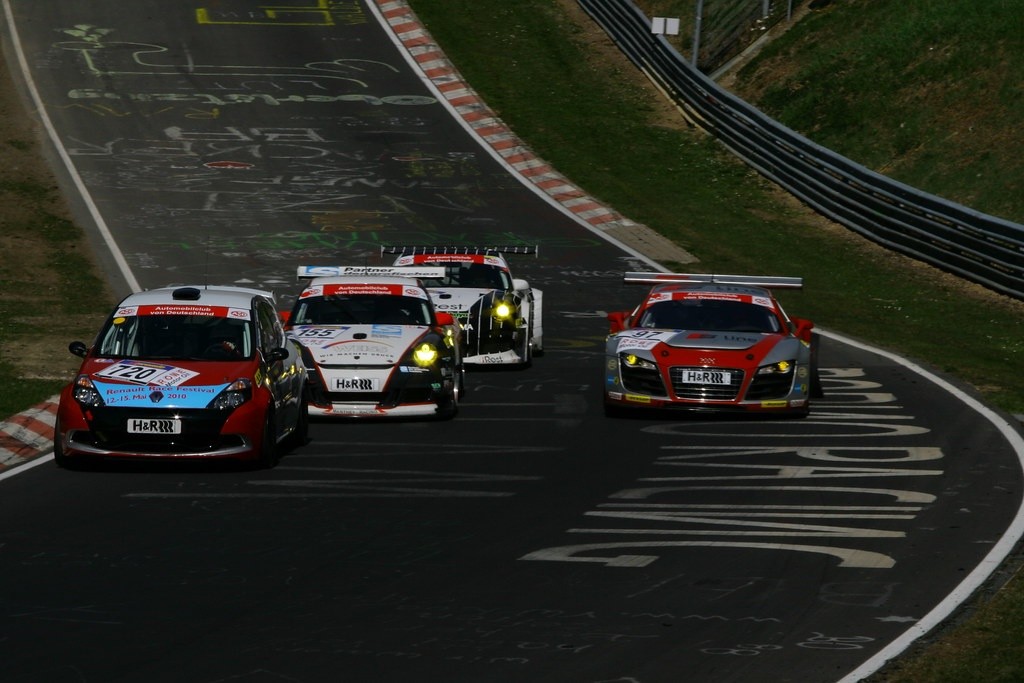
[378,244,545,365]
[275,267,464,421]
[53,285,309,468]
[603,270,827,419]
